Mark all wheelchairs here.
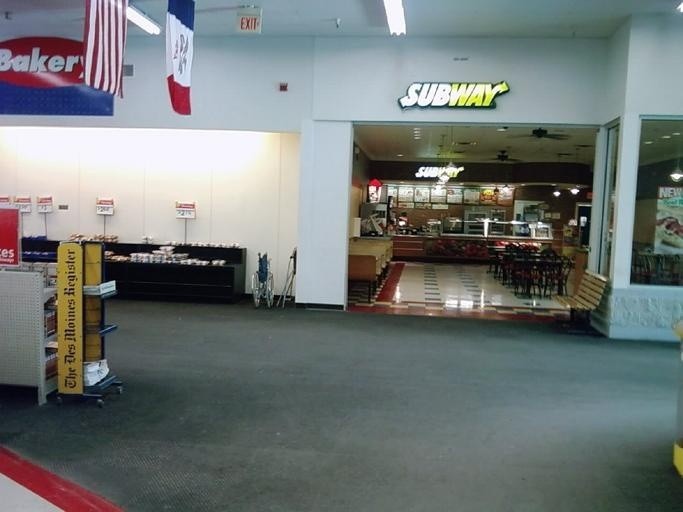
[251,251,274,309]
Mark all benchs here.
[348,237,393,304]
[551,269,608,337]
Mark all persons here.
[538,242,557,282]
[398,211,409,223]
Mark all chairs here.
[486,239,572,301]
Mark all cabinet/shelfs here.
[59,239,123,399]
[2,260,58,408]
[21,238,245,304]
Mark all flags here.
[77,0,129,98]
[164,0,194,116]
